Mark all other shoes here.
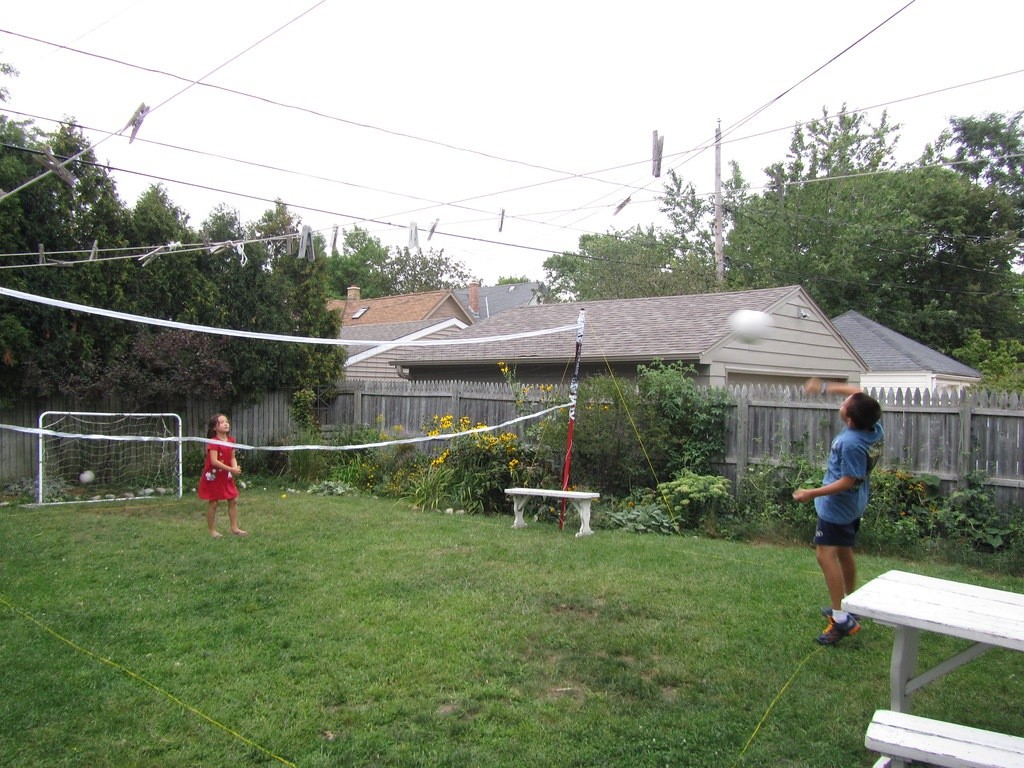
[211,533,222,538]
[231,529,247,537]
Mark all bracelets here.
[820,382,827,395]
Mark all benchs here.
[864,709,1024,768]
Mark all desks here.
[840,568,1024,717]
[504,487,600,538]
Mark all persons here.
[198,413,248,538]
[792,377,885,645]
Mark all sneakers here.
[820,606,861,620]
[815,614,861,646]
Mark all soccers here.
[727,307,773,344]
[79,469,95,483]
